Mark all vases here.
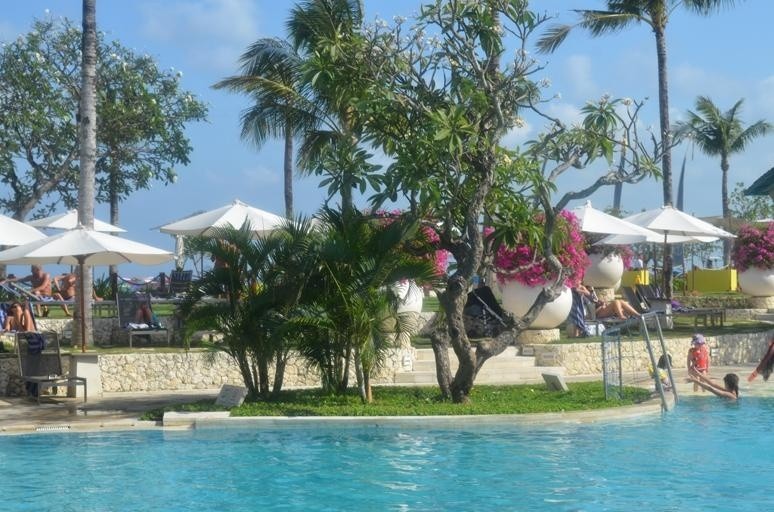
[377,277,423,321]
[736,263,774,296]
[493,275,573,328]
[580,249,624,288]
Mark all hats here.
[692,333,705,345]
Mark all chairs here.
[113,291,168,347]
[0,280,75,344]
[12,330,87,404]
[579,281,727,336]
[169,270,192,297]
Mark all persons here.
[209,237,229,304]
[687,333,711,392]
[650,353,673,392]
[570,284,592,337]
[573,276,643,319]
[0,264,72,318]
[683,366,740,402]
[3,302,36,333]
[227,240,242,303]
[135,304,159,328]
[54,264,104,302]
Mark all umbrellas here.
[159,196,303,242]
[0,214,49,246]
[25,207,128,234]
[568,198,738,298]
[0,229,174,352]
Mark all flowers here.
[585,232,634,271]
[483,208,591,288]
[361,205,449,289]
[728,221,774,272]
